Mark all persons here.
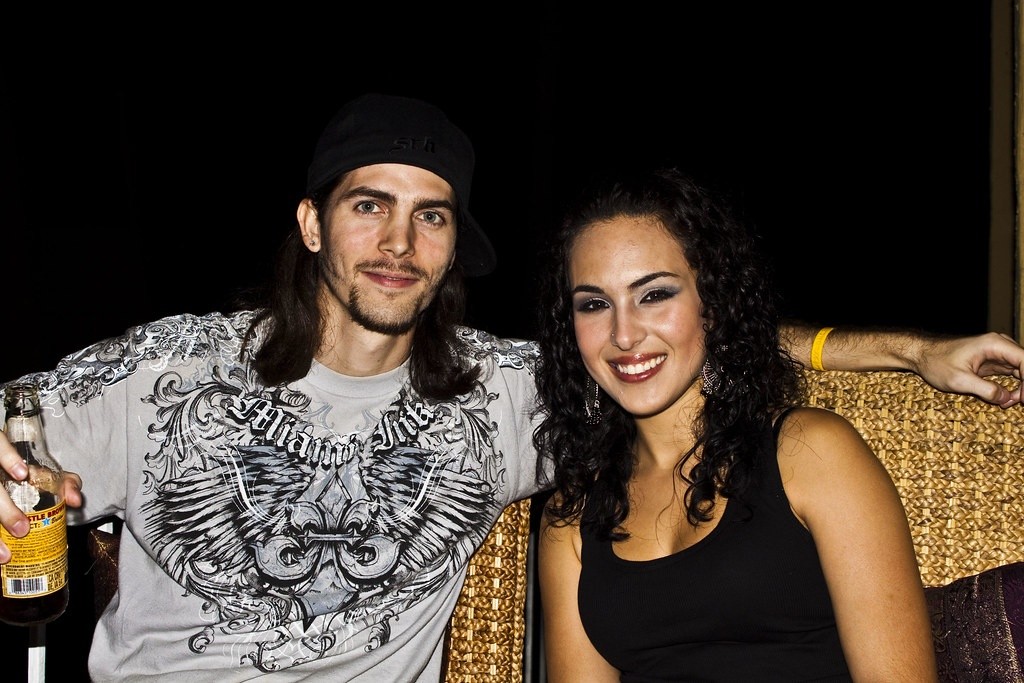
[1,97,1024,682]
[537,170,937,683]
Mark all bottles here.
[0,382,69,626]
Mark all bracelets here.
[812,327,835,371]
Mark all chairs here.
[88,369,1024,683]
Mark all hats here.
[307,94,496,276]
